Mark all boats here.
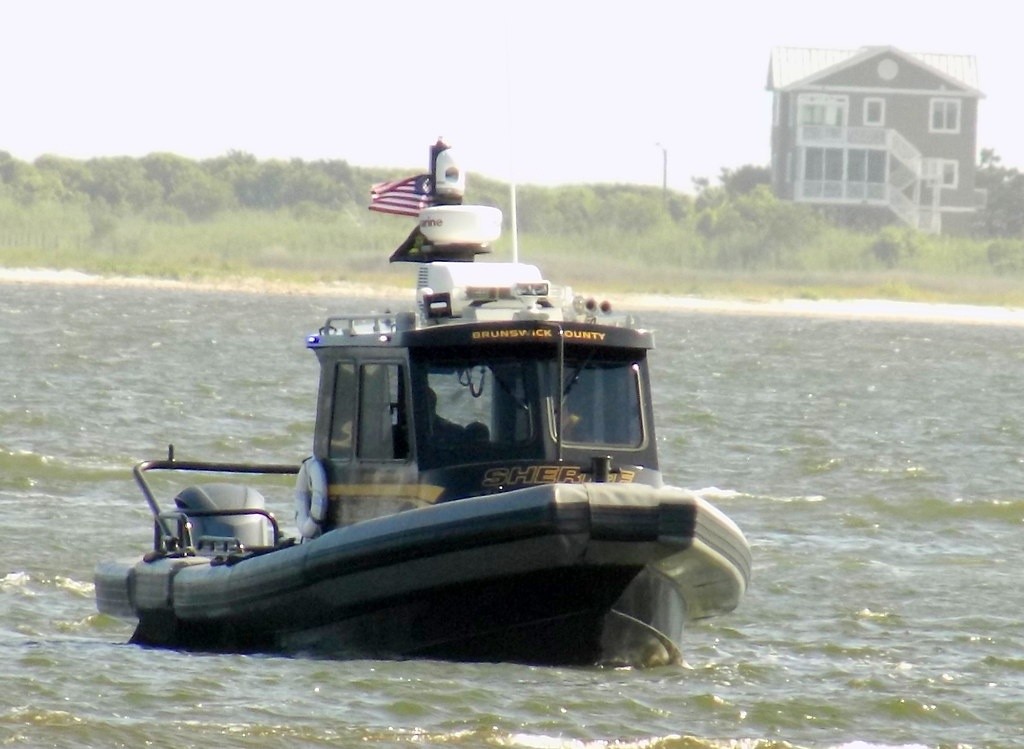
[90,139,753,670]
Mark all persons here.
[393,391,464,460]
[465,423,490,442]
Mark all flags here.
[369,174,436,216]
